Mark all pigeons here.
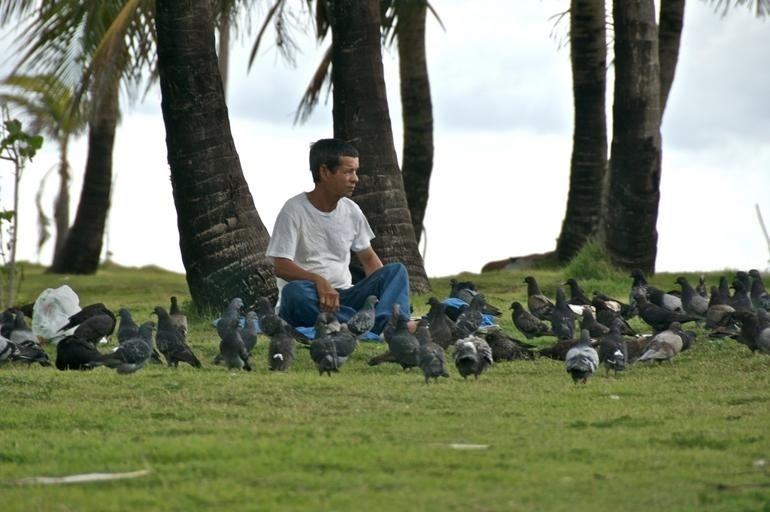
[0,266,769,386]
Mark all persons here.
[265,138,420,341]
